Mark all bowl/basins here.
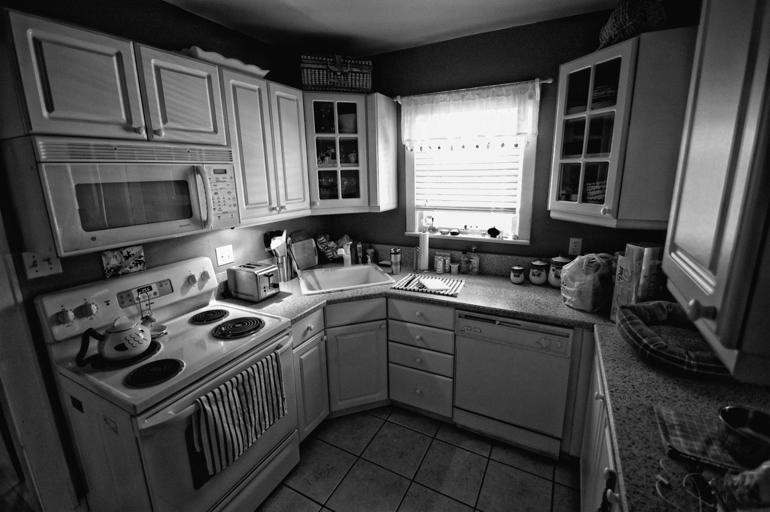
[718,401,770,466]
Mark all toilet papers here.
[418,231,429,270]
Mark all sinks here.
[299,264,395,295]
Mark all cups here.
[391,248,402,275]
[510,255,573,288]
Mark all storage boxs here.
[598,0,673,50]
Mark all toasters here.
[226,262,280,303]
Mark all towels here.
[191,350,288,476]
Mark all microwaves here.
[14,138,242,263]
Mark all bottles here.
[434,245,480,275]
[357,242,375,263]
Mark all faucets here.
[337,241,353,265]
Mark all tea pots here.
[73,315,156,367]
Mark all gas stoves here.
[54,301,291,416]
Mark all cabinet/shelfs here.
[324,296,389,419]
[388,297,455,424]
[220,68,311,225]
[1,8,227,147]
[302,90,370,217]
[661,1,769,385]
[545,34,641,220]
[291,307,331,444]
[579,342,624,512]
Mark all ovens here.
[136,337,299,512]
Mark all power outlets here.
[20,249,65,281]
[568,237,582,256]
